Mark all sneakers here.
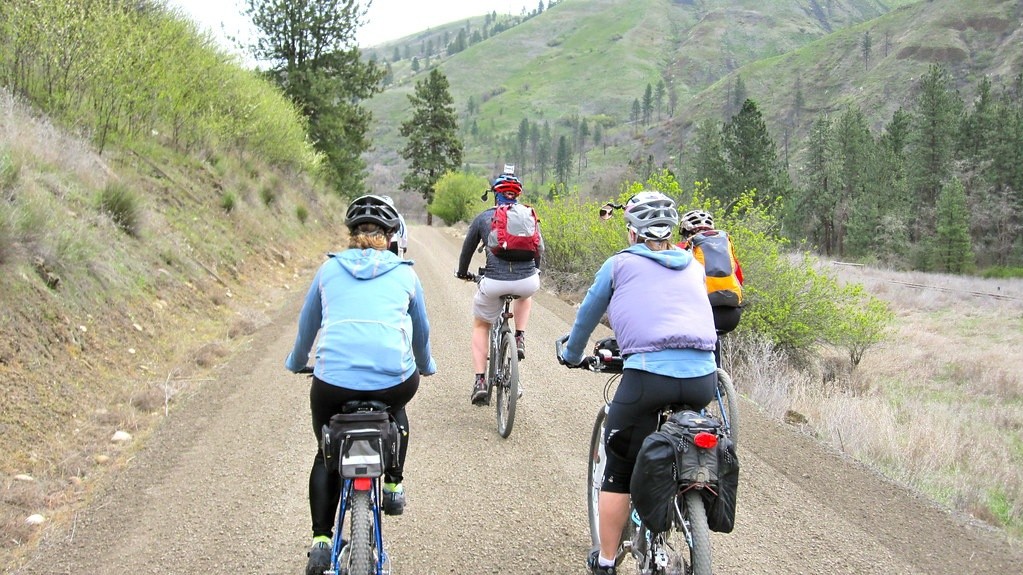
[585,545,617,575]
[306,541,331,575]
[383,488,406,516]
[471,378,488,404]
[516,336,525,359]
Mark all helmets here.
[623,192,678,240]
[345,195,401,233]
[679,210,714,235]
[492,173,523,194]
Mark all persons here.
[288,195,435,575]
[456,171,546,402]
[674,210,745,399]
[562,190,719,575]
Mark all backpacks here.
[484,203,539,263]
[684,230,742,306]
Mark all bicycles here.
[293,366,424,575]
[455,272,524,437]
[556,328,738,575]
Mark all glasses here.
[601,210,613,216]
